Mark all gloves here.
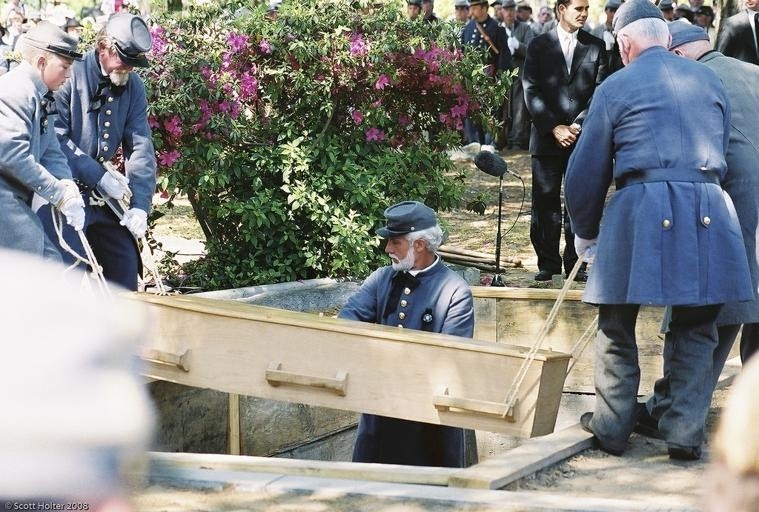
[54,179,87,232]
[574,232,598,262]
[119,207,148,238]
[507,36,520,56]
[100,170,129,201]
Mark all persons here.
[628,21,759,442]
[521,0,609,285]
[0,242,167,512]
[718,0,759,65]
[28,10,158,295]
[0,1,143,79]
[589,0,718,55]
[0,20,87,265]
[408,0,553,154]
[334,197,477,471]
[562,0,759,461]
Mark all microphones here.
[474,150,521,177]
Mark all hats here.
[375,200,436,241]
[604,0,715,51]
[24,19,86,62]
[455,0,532,14]
[107,12,153,69]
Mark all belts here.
[617,166,724,187]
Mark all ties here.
[754,13,758,54]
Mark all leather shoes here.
[581,412,624,457]
[667,442,702,460]
[535,269,559,281]
[566,272,588,282]
[633,402,659,436]
